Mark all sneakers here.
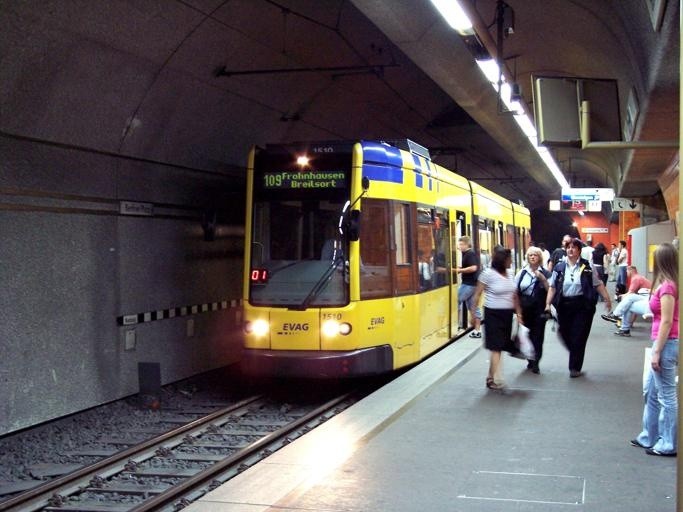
[614,330,631,336]
[646,448,677,457]
[628,439,651,448]
[601,313,618,323]
[469,331,481,338]
[570,369,580,377]
[528,360,540,374]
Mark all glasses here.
[571,274,573,282]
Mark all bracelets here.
[516,313,524,318]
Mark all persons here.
[318,223,363,261]
[470,247,525,390]
[417,250,449,291]
[626,266,652,329]
[601,292,652,337]
[538,234,629,302]
[544,238,612,378]
[513,245,553,374]
[629,242,678,457]
[457,235,483,338]
[487,244,511,273]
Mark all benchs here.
[640,313,655,320]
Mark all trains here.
[236,139,532,380]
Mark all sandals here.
[487,377,497,388]
